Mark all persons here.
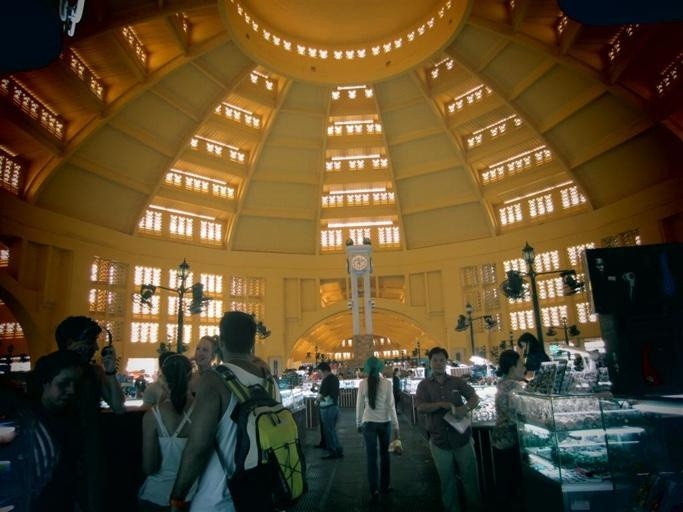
[594,253,605,271]
[0,312,552,512]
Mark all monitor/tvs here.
[581,242,683,395]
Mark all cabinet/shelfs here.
[309,362,411,408]
[411,351,680,510]
[99,352,308,491]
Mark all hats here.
[363,356,384,373]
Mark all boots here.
[319,446,344,460]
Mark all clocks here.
[351,256,367,270]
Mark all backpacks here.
[199,365,309,512]
